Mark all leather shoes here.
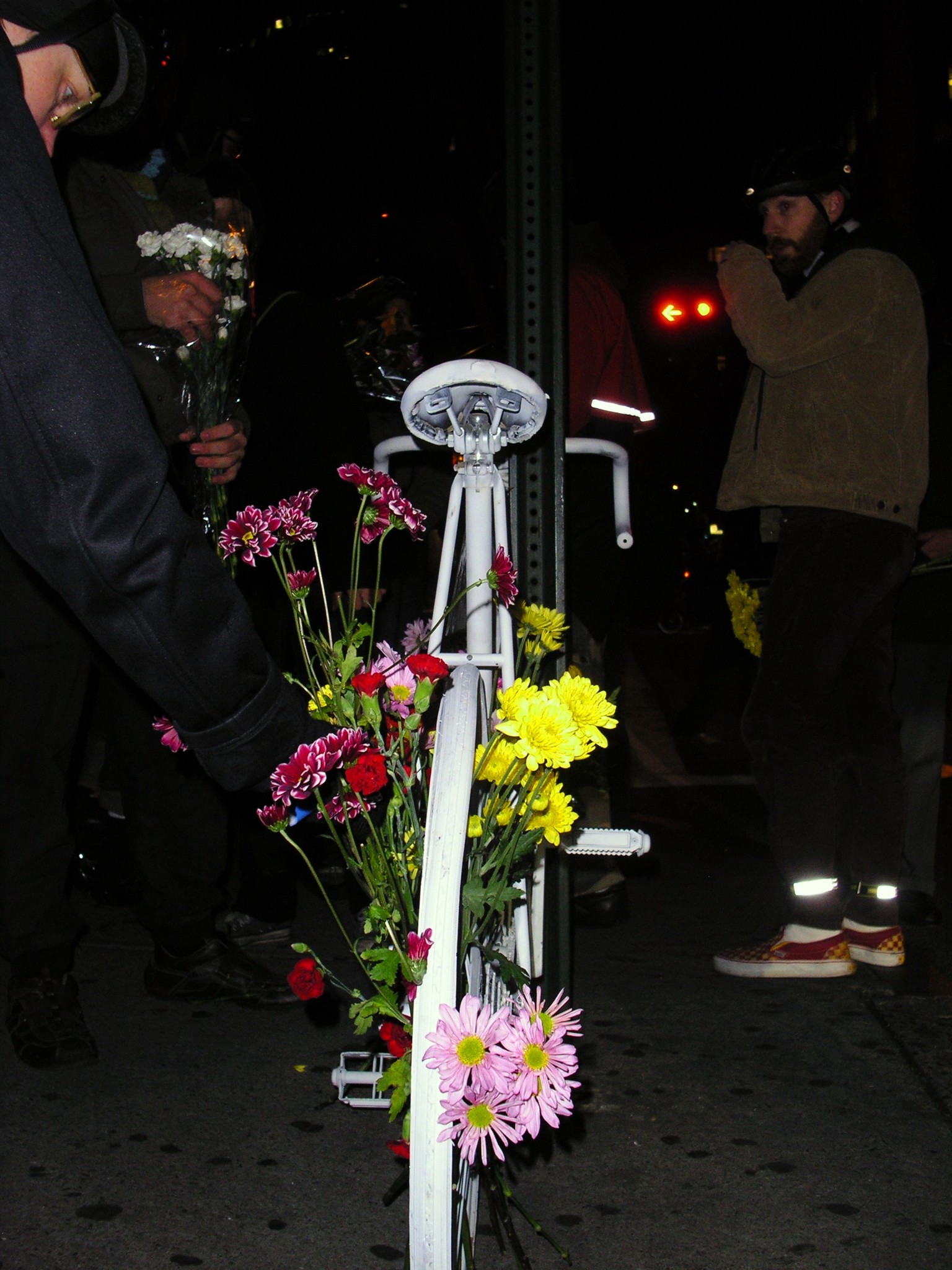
[6,969,99,1069]
[143,935,300,1002]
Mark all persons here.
[564,227,655,928]
[0,0,437,1074]
[713,140,929,977]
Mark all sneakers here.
[841,914,904,966]
[713,924,856,978]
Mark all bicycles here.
[331,355,652,1270]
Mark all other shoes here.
[316,864,349,887]
[569,870,629,927]
[222,915,301,980]
[631,786,715,840]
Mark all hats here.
[752,143,857,200]
[0,0,150,139]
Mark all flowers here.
[150,461,622,1269]
[137,218,253,572]
[723,569,765,659]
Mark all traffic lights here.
[660,300,683,324]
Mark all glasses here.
[37,43,102,131]
